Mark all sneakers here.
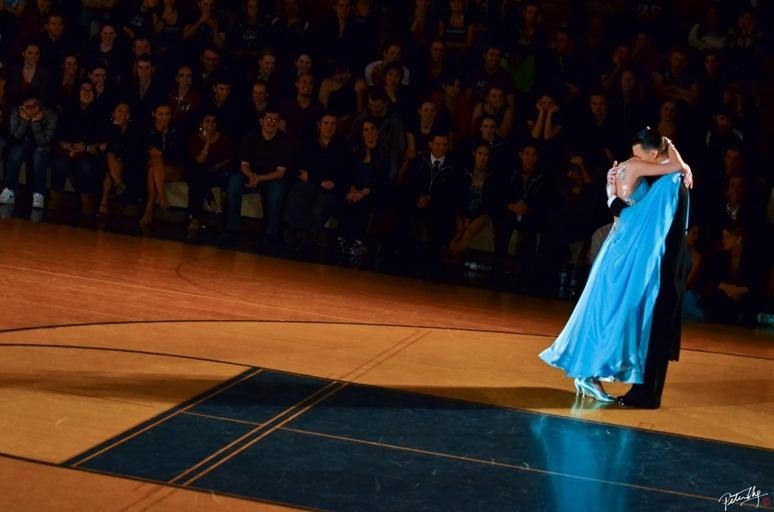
[0,187,19,205]
[31,191,46,209]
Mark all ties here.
[430,159,441,179]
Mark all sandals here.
[95,204,111,221]
[112,182,127,195]
[157,197,171,210]
[139,213,156,225]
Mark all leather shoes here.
[616,394,662,410]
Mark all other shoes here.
[184,203,519,281]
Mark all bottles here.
[758,313,774,323]
[558,269,577,301]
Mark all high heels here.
[574,374,617,402]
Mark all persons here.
[0,0,774,328]
[616,124,691,408]
[537,131,694,403]
